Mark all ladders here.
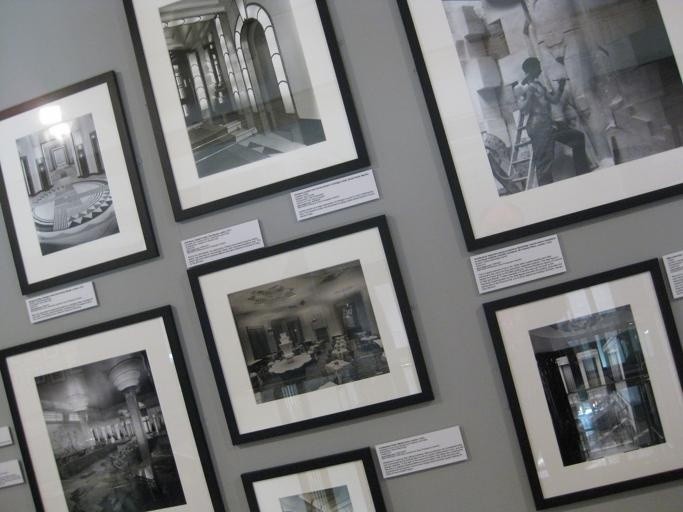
[504,110,542,192]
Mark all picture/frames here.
[186,215,435,445]
[397,0,683,252]
[0,71,160,295]
[0,304,225,512]
[240,446,387,512]
[483,257,683,511]
[123,0,371,222]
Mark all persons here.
[514,58,593,185]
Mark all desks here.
[246,330,388,398]
